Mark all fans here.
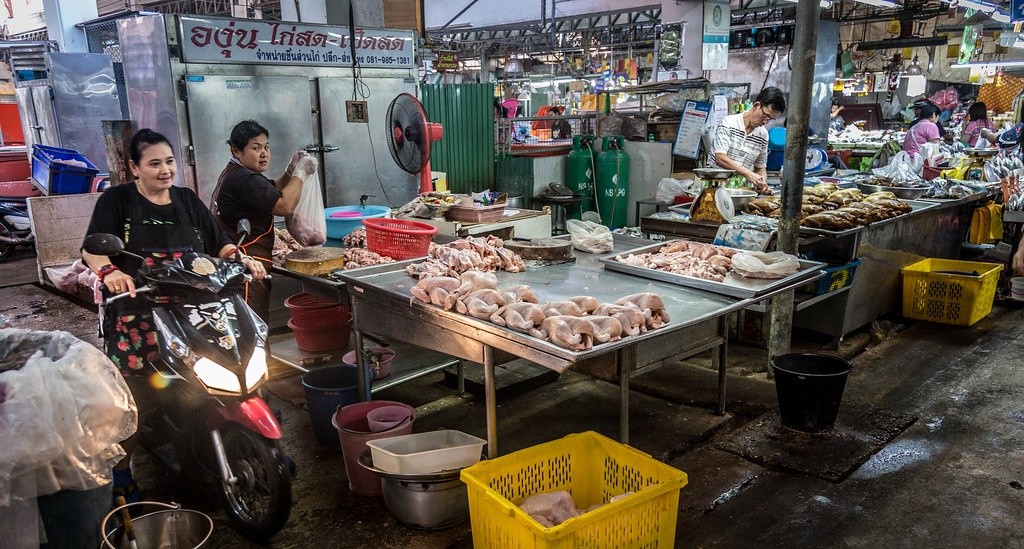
[386,92,443,192]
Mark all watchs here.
[98,265,119,283]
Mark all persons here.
[80,126,267,516]
[959,101,996,147]
[830,104,845,132]
[908,97,954,141]
[784,116,848,169]
[549,107,572,139]
[902,105,941,163]
[492,97,514,154]
[980,100,1024,165]
[707,86,787,195]
[209,120,317,420]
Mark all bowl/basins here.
[332,212,362,218]
[725,188,758,211]
[854,178,934,200]
[767,126,786,150]
[344,348,396,378]
[324,205,391,238]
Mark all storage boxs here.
[799,257,862,296]
[30,144,99,197]
[900,258,1005,326]
[569,81,585,92]
[366,429,487,472]
[459,431,687,549]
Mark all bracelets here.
[242,255,255,260]
[984,130,992,138]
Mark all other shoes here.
[261,389,270,400]
[268,405,281,418]
[112,469,141,510]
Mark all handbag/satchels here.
[285,168,326,247]
[872,150,921,186]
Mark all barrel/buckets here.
[101,500,213,549]
[770,352,852,434]
[506,194,525,209]
[1009,278,1024,301]
[767,151,784,171]
[296,364,489,530]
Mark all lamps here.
[781,0,1024,77]
[501,29,617,101]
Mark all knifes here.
[512,237,546,243]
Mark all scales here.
[688,168,736,223]
[804,135,835,177]
[963,149,1000,182]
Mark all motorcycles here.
[0,179,47,262]
[83,217,294,541]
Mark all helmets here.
[908,98,933,109]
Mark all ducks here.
[747,183,913,224]
[412,271,668,355]
[616,240,737,281]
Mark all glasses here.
[761,106,775,122]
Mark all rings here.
[261,267,264,268]
[114,286,121,290]
[256,266,260,268]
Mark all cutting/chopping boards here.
[503,238,572,261]
[284,247,345,276]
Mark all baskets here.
[362,217,439,261]
[284,292,353,352]
[445,204,506,223]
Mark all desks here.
[529,194,590,235]
[635,197,671,228]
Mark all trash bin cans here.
[0,326,115,549]
